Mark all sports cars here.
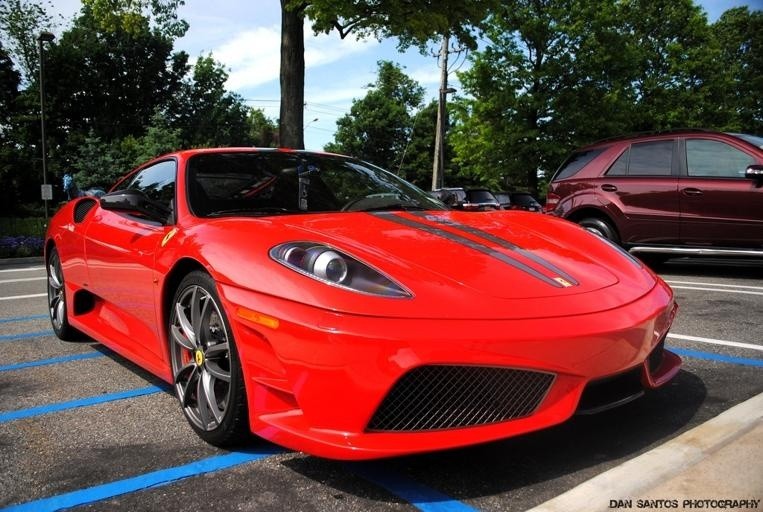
[41,143,689,463]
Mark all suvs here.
[422,185,547,215]
[541,130,763,264]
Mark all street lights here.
[35,29,57,232]
